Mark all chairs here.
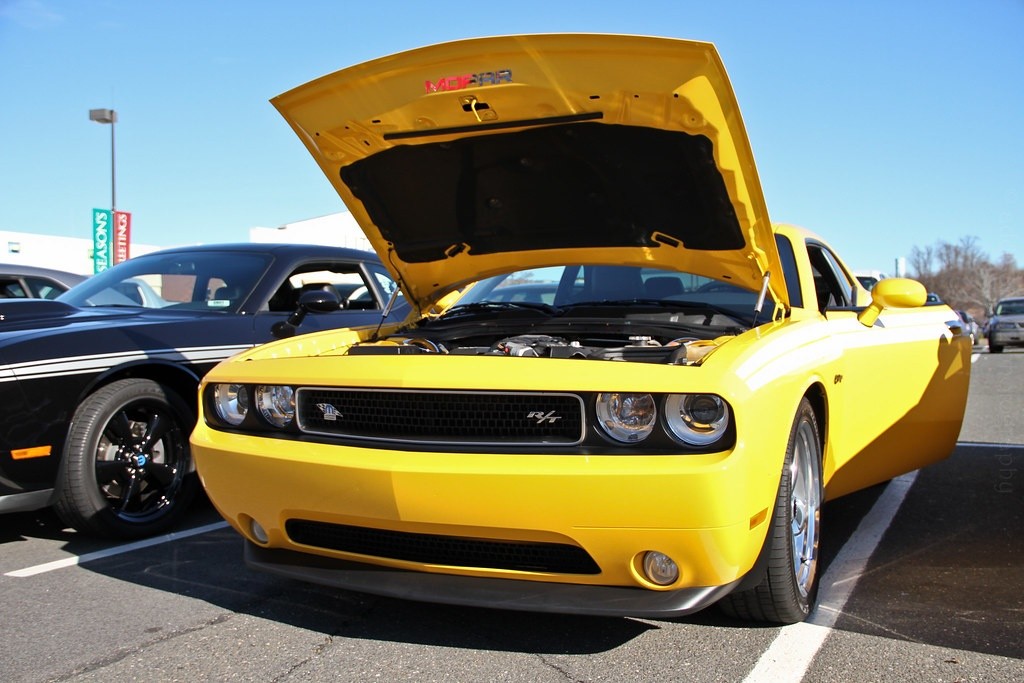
[522,293,544,304]
[296,283,342,311]
[644,277,685,298]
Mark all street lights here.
[88,107,119,269]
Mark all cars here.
[983,297,1024,353]
[0,264,142,307]
[0,241,437,552]
[958,311,980,345]
[187,31,974,626]
